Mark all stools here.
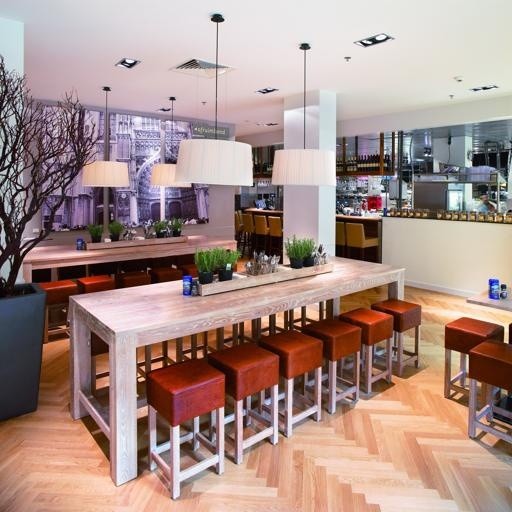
[444,317,511,444]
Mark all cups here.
[183,276,192,295]
[77,239,82,250]
[346,209,350,216]
[489,279,500,299]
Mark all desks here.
[21,234,238,285]
[466,286,511,312]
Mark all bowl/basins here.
[367,213,379,218]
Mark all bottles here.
[361,198,368,217]
[270,193,276,209]
[337,155,390,172]
[353,197,358,213]
[500,284,507,298]
[82,241,85,250]
[191,282,197,296]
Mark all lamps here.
[175,14,254,187]
[83,86,129,188]
[150,96,192,188]
[271,43,337,186]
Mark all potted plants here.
[0,54,105,422]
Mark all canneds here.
[389,207,430,217]
[436,209,512,223]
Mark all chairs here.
[235,210,379,260]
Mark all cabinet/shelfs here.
[252,133,395,178]
